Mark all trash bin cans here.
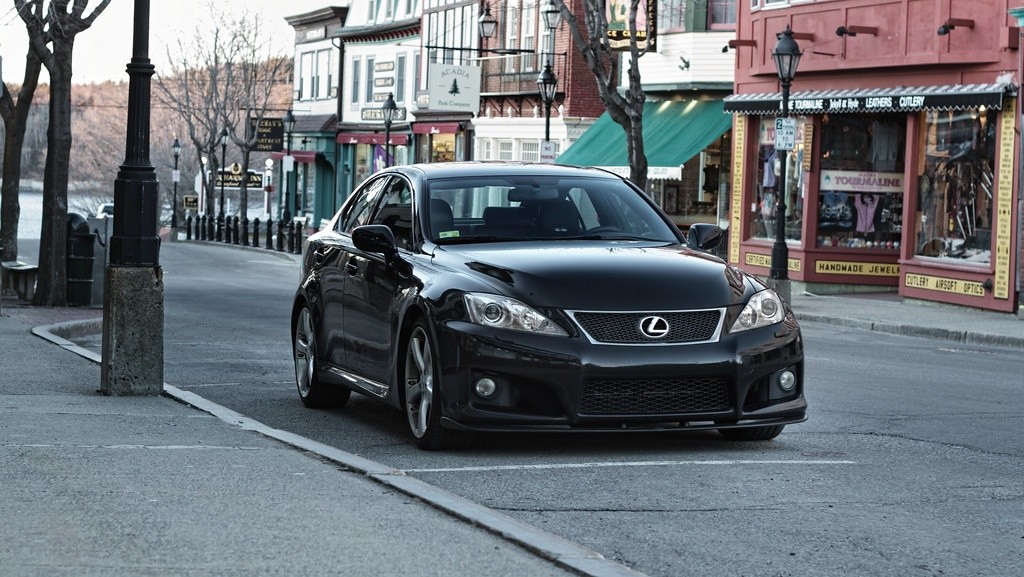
[687,224,728,263]
[64,212,96,307]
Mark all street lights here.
[170,139,181,241]
[218,128,230,241]
[281,109,296,250]
[536,61,557,142]
[764,22,805,305]
[381,91,398,167]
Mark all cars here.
[96,201,115,220]
[291,161,807,453]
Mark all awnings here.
[554,100,734,181]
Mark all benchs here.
[452,206,534,240]
[0,259,40,303]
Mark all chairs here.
[531,201,581,238]
[410,198,456,245]
